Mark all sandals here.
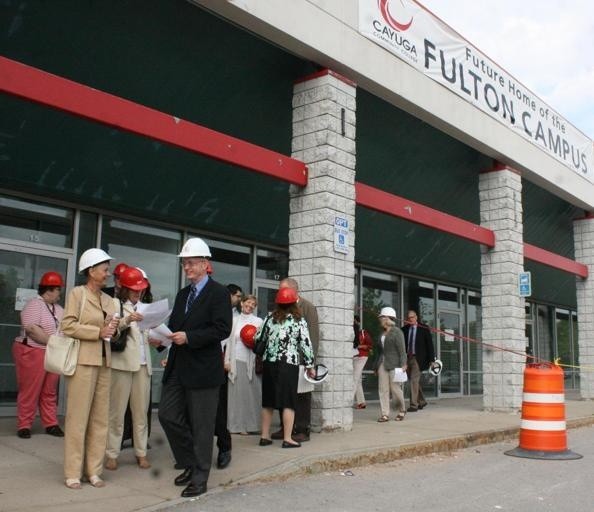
[66,456,150,489]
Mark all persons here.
[13,237,320,497]
[352,307,434,423]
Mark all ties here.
[409,326,413,358]
[188,288,195,311]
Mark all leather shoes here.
[260,429,309,447]
[46,425,64,436]
[218,450,231,469]
[357,404,366,409]
[17,428,30,438]
[175,463,206,496]
[378,402,427,422]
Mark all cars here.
[563,367,571,379]
[422,368,469,388]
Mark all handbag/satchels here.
[253,339,266,374]
[44,335,80,375]
[111,327,130,352]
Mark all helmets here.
[377,307,396,318]
[240,325,257,348]
[428,360,442,376]
[177,238,212,258]
[79,249,148,291]
[40,272,63,286]
[275,288,298,304]
[304,364,328,383]
